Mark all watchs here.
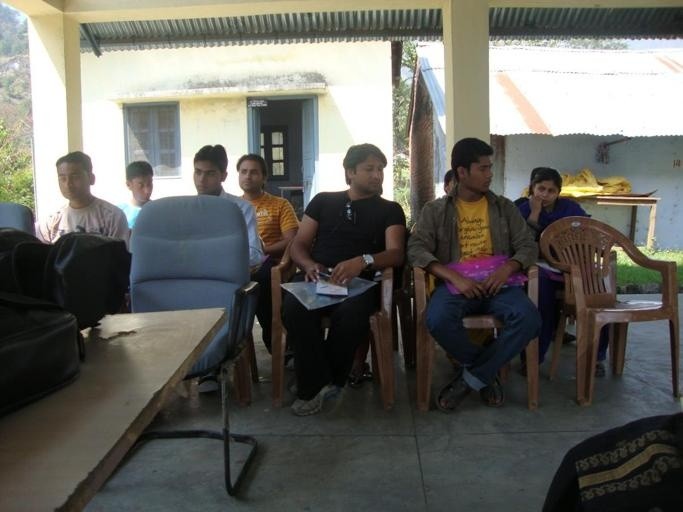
[362,251,375,266]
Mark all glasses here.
[344,201,354,222]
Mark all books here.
[316,276,348,297]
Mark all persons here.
[511,166,610,378]
[34,152,130,254]
[345,334,373,389]
[406,137,543,414]
[114,161,153,230]
[281,144,407,416]
[443,167,458,195]
[512,167,545,208]
[236,154,300,356]
[193,145,263,276]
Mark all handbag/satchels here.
[0,290,85,418]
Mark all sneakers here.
[199,376,219,393]
[289,380,337,415]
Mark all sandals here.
[480,376,505,407]
[436,371,471,414]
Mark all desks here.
[0,305,231,512]
[578,193,661,251]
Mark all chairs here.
[412,265,541,414]
[126,194,259,499]
[268,244,396,414]
[0,200,37,237]
[539,213,683,408]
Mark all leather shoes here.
[348,370,373,388]
